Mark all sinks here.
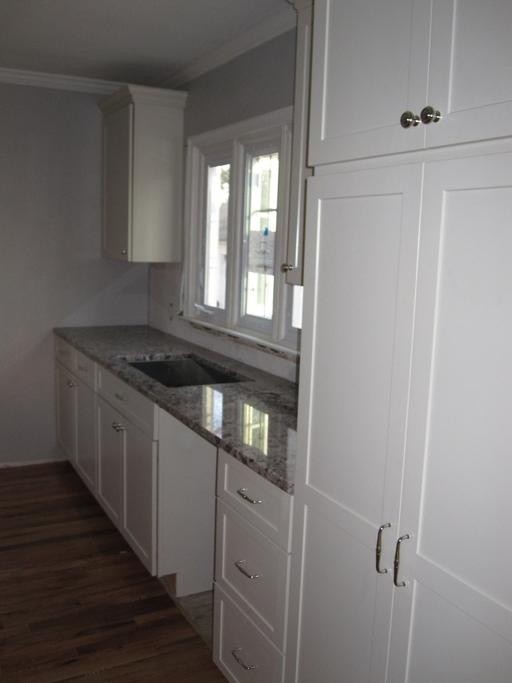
[116,349,256,389]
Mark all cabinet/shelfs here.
[98,365,217,602]
[286,1,511,683]
[52,337,99,501]
[98,85,188,262]
[212,445,294,683]
